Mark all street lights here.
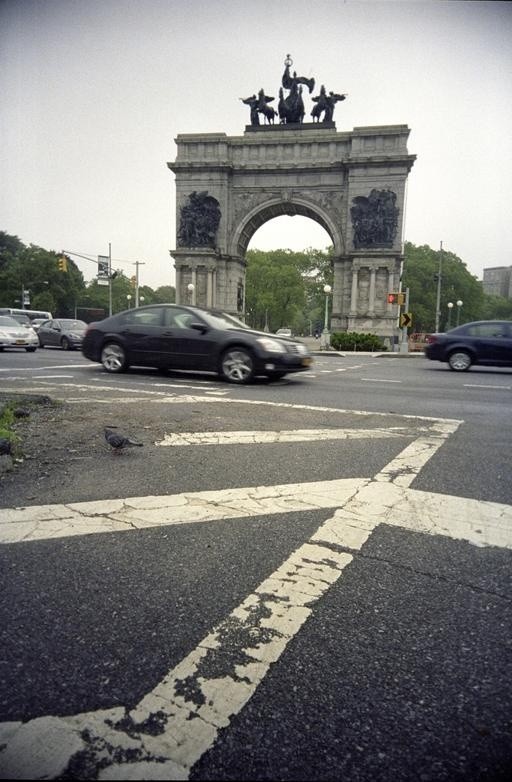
[448,302,453,329]
[322,285,331,333]
[188,284,195,305]
[126,295,145,311]
[457,300,463,327]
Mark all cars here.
[82,303,312,384]
[0,308,88,352]
[424,320,512,372]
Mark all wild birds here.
[102,428,144,453]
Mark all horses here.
[310,85,334,123]
[251,84,305,125]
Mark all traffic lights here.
[388,294,394,304]
[57,259,67,273]
